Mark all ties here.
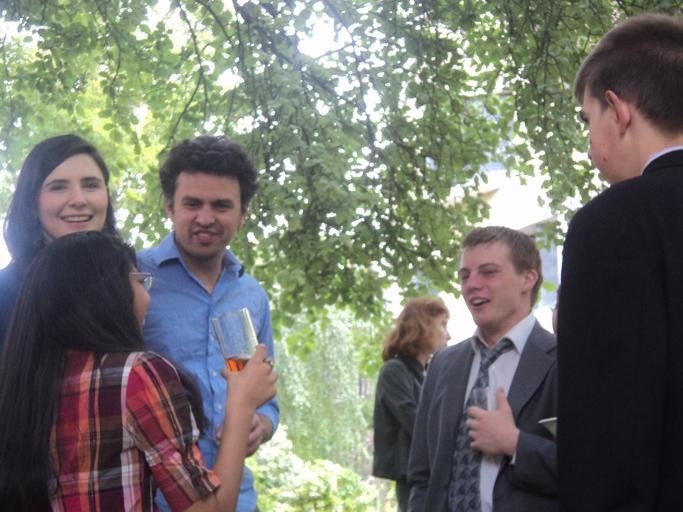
[447,337,514,511]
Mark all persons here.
[0,229,278,510]
[0,134,116,349]
[550,11,682,511]
[367,294,452,511]
[130,131,278,511]
[404,226,563,512]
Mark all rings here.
[262,358,275,368]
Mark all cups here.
[211,307,259,373]
[473,386,502,412]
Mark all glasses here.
[128,272,154,291]
